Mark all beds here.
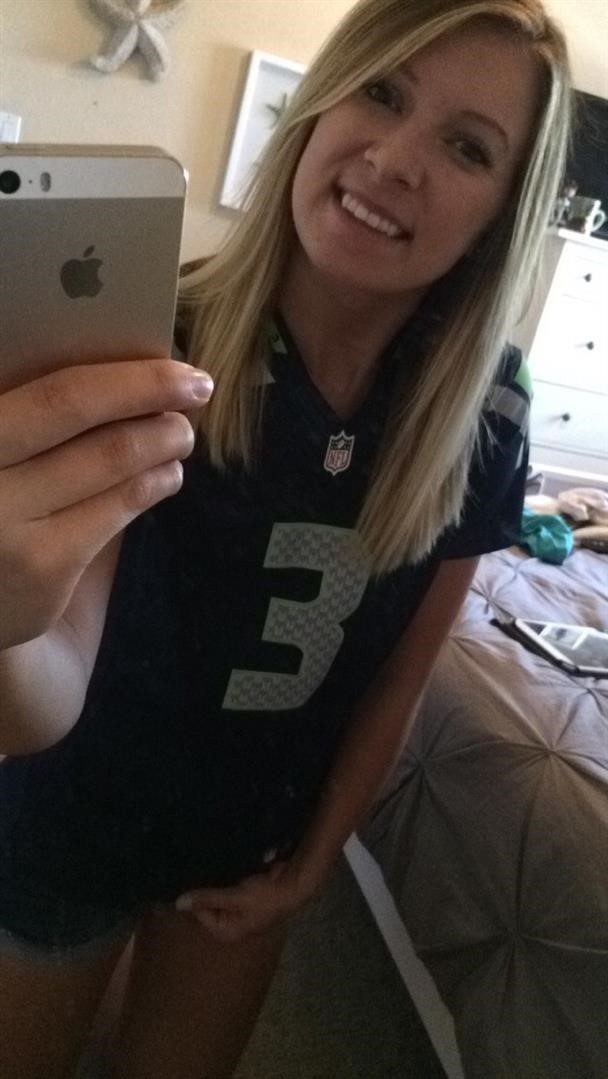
[341,483,608,1079]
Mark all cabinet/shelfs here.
[507,224,607,480]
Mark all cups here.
[563,196,607,233]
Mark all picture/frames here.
[217,50,303,214]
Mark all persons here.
[0,0,571,1079]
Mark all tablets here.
[514,616,608,675]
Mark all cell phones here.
[0,142,187,393]
